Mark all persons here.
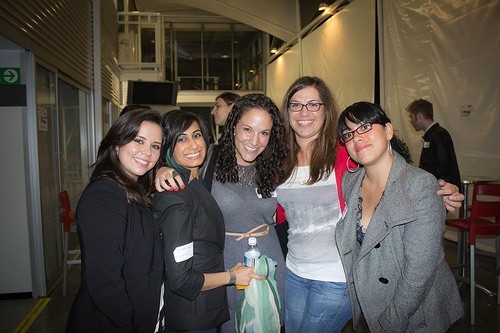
[408,99,460,197]
[151,92,289,333]
[64,105,168,332]
[145,111,267,333]
[333,102,466,332]
[269,74,465,332]
[209,93,241,129]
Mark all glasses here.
[287,103,324,112]
[339,121,379,144]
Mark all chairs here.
[58,190,82,296]
[444,182,500,326]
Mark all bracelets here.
[225,269,236,284]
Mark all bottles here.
[244,237,261,268]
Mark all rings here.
[172,170,179,178]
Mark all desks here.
[440,176,496,286]
